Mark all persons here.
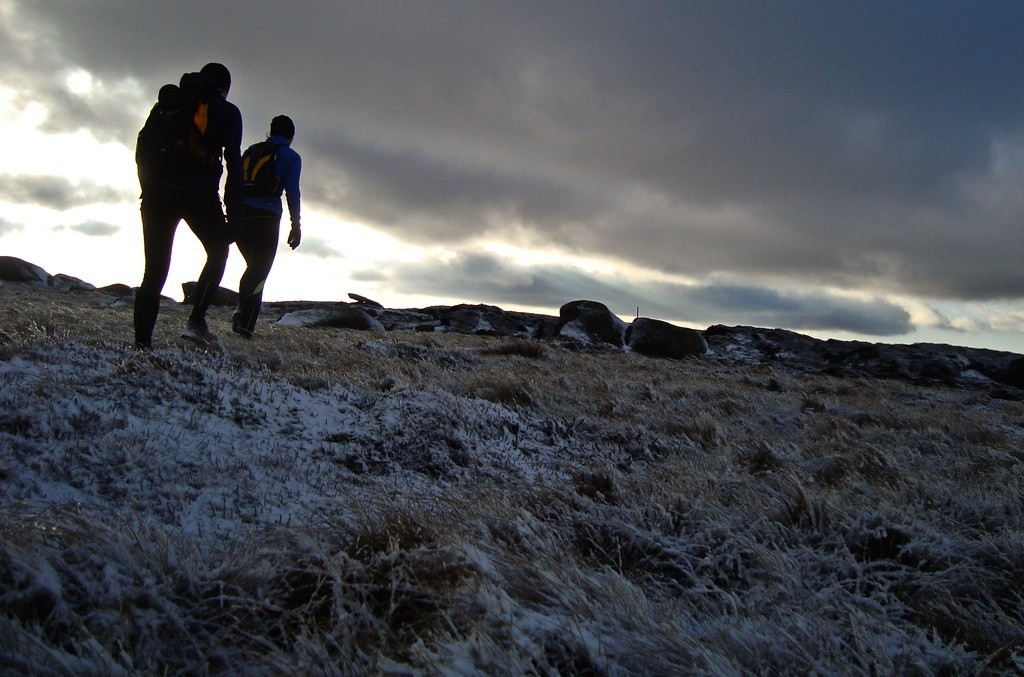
[134,63,243,352]
[225,115,302,339]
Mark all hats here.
[200,62,231,92]
[270,115,295,139]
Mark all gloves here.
[287,223,301,250]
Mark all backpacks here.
[151,86,208,177]
[239,142,285,202]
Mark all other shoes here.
[231,322,255,338]
[180,323,218,346]
[134,338,154,352]
